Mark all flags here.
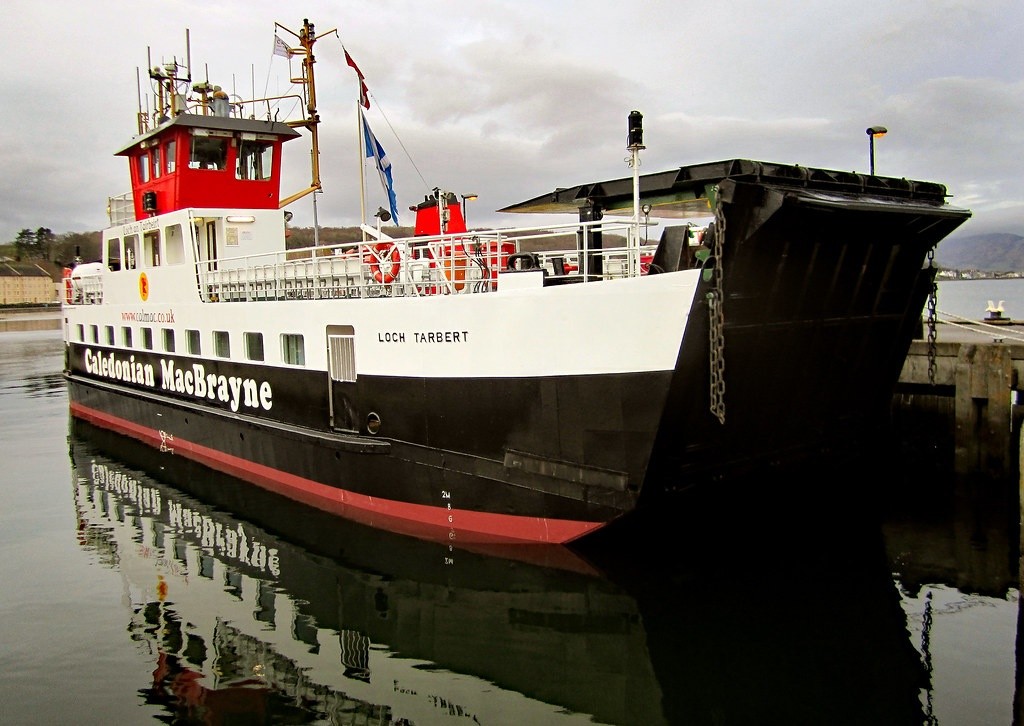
[273,35,294,59]
[361,112,399,228]
[344,48,371,111]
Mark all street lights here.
[461,193,478,224]
[866,126,887,176]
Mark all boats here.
[56,16,975,584]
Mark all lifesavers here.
[369,241,400,285]
